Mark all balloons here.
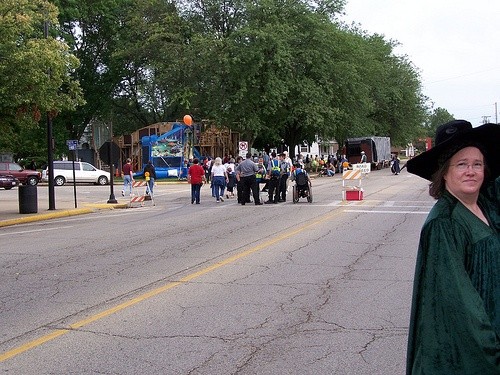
[183,115,192,126]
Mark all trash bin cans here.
[18,185,38,214]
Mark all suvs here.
[0,159,41,189]
[42,161,110,185]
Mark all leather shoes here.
[238,199,288,205]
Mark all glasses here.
[449,161,486,171]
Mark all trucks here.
[346,136,393,170]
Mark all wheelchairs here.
[292,170,314,203]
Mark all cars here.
[0,172,19,190]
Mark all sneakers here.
[216,193,234,203]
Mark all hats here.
[406,120,500,184]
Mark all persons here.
[121,158,135,196]
[389,155,401,175]
[143,160,156,194]
[360,151,367,163]
[184,150,353,205]
[406,120,500,375]
[30,158,36,170]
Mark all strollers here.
[318,165,328,177]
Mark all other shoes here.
[122,190,125,196]
[129,194,134,198]
[196,203,200,204]
[191,201,194,204]
